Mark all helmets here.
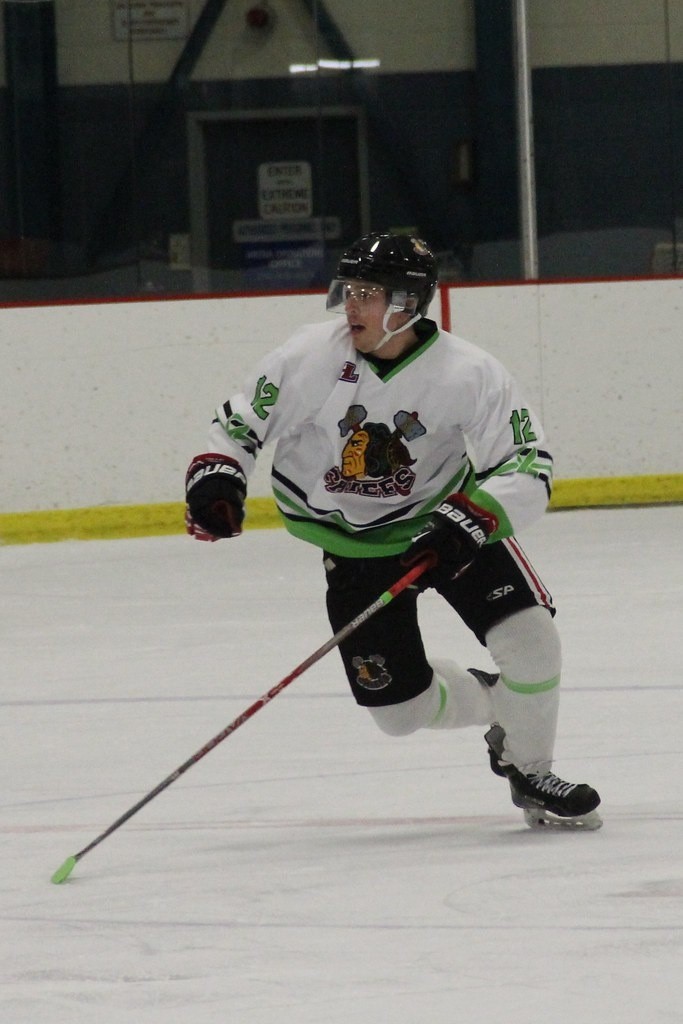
[332,230,440,315]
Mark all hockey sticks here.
[51,555,440,886]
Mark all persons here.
[184,230,603,832]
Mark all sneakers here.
[467,668,508,778]
[502,762,604,831]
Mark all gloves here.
[188,462,248,538]
[400,493,499,589]
